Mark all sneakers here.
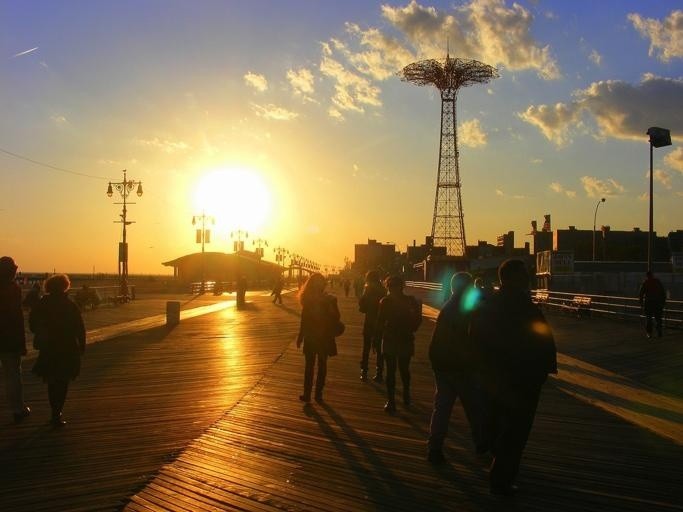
[54,410,66,427]
[490,486,518,495]
[14,407,31,424]
[360,375,382,382]
[385,399,396,413]
[299,396,306,400]
[427,448,443,461]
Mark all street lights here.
[106,169,142,295]
[229,227,320,289]
[645,123,672,302]
[591,197,605,261]
[191,209,216,294]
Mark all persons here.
[200,272,351,307]
[639,271,667,340]
[427,270,489,464]
[296,272,345,404]
[23,275,102,311]
[358,269,387,384]
[0,256,28,424]
[467,259,558,496]
[28,273,86,428]
[373,275,421,415]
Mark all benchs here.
[531,292,593,321]
